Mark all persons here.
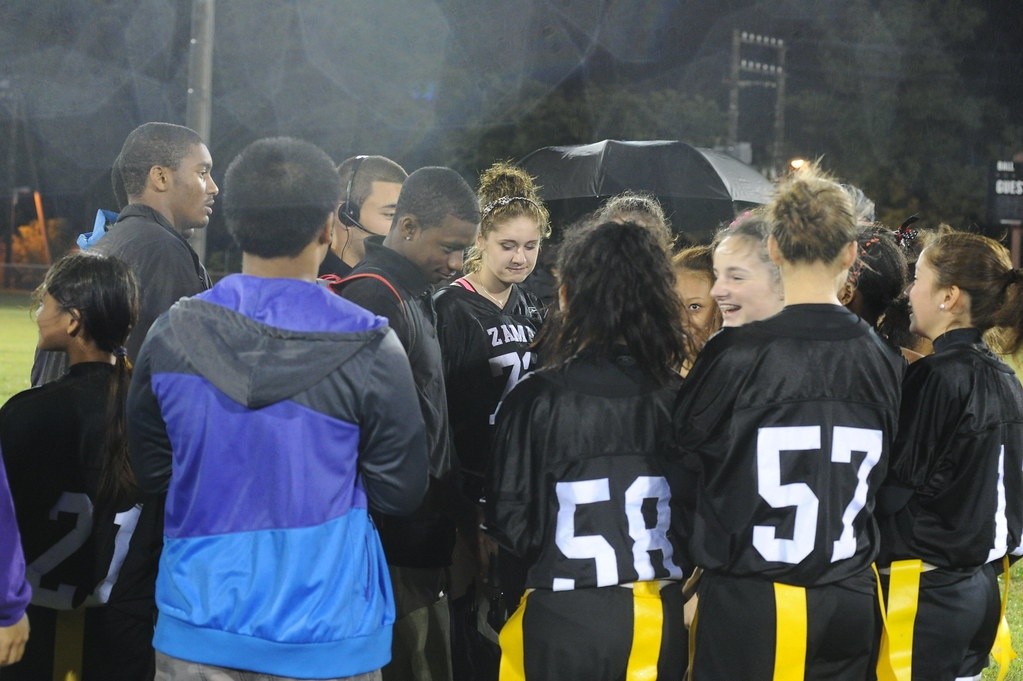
[481,220,707,681]
[0,447,32,667]
[0,253,138,681]
[25,122,218,680]
[599,199,672,254]
[346,166,482,681]
[710,209,784,327]
[842,210,1023,681]
[672,176,909,681]
[433,165,550,681]
[671,245,723,377]
[317,155,409,296]
[125,134,429,681]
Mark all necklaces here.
[479,279,512,306]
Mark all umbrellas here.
[521,138,780,207]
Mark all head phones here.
[337,157,366,227]
